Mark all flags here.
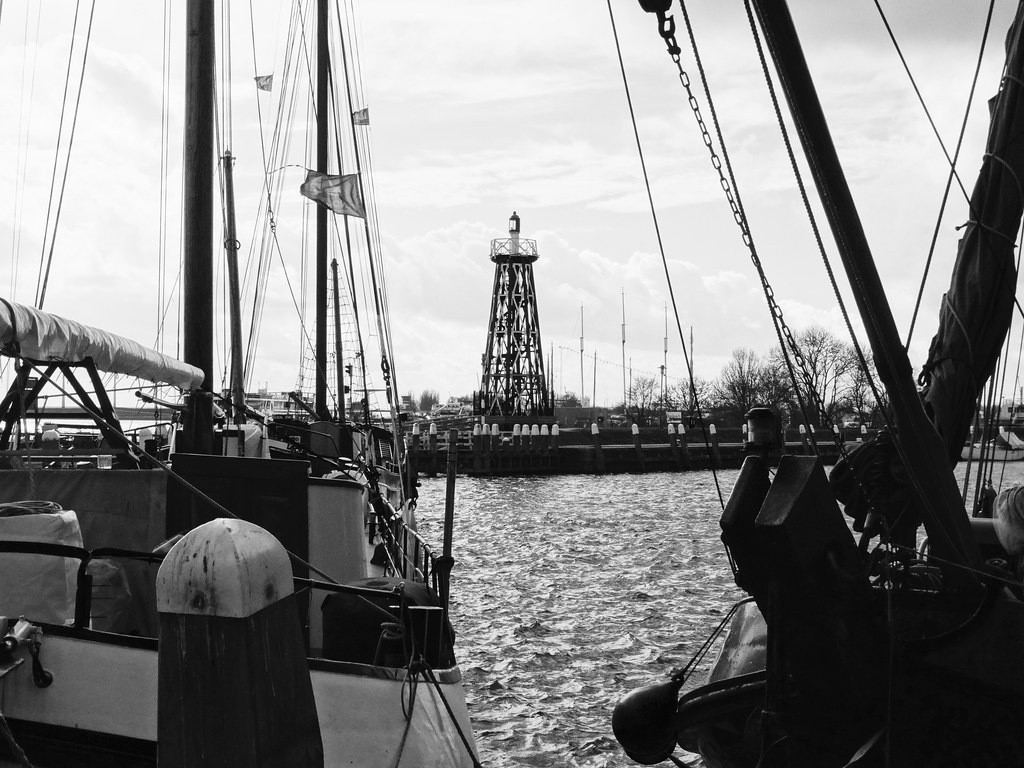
[298,166,366,216]
[349,109,372,126]
[254,74,274,92]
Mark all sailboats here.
[1,0,490,767]
[594,0,1024,768]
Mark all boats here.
[960,423,1024,462]
